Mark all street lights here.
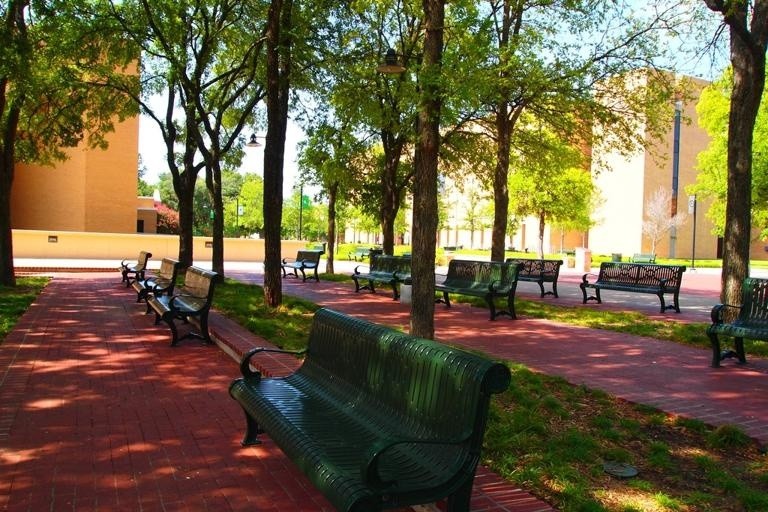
[246,134,266,147]
[292,183,305,241]
[230,197,238,232]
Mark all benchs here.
[706,278,768,368]
[227,306,512,512]
[280,251,323,283]
[580,262,686,314]
[117,250,219,347]
[349,254,564,321]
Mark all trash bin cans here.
[369,249,383,272]
[575,247,592,272]
[612,254,621,262]
